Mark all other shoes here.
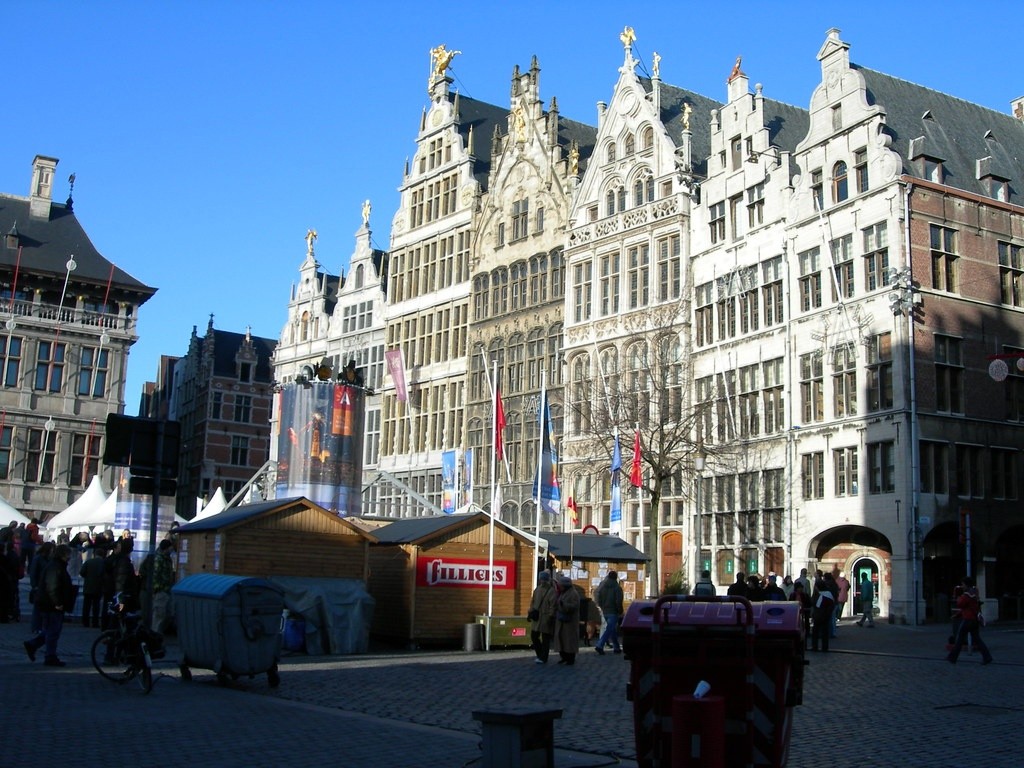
[856,622,863,627]
[867,625,875,628]
[945,655,957,664]
[24,641,36,661]
[614,649,621,653]
[557,658,565,664]
[534,659,543,663]
[983,657,992,664]
[595,647,605,654]
[45,660,66,667]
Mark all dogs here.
[579,619,603,646]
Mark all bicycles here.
[90,590,166,692]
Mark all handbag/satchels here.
[557,612,571,622]
[64,585,80,613]
[978,613,986,627]
[528,610,539,621]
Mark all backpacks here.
[136,551,157,597]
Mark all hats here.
[539,572,550,581]
[560,578,572,587]
[160,540,171,550]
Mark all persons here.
[430,44,452,68]
[764,575,788,601]
[782,575,795,600]
[727,572,749,597]
[21,531,173,665]
[693,570,716,595]
[949,576,994,665]
[653,51,661,77]
[526,570,555,664]
[0,518,44,623]
[569,148,581,174]
[362,200,372,223]
[595,571,624,654]
[808,569,850,652]
[789,582,812,608]
[619,26,637,45]
[796,568,811,597]
[680,101,692,132]
[551,576,581,666]
[856,573,875,628]
[304,228,317,253]
[746,575,768,601]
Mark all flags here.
[631,430,642,488]
[567,484,579,525]
[495,386,507,461]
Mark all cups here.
[695,680,710,698]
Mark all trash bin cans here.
[170,573,285,684]
[616,594,809,768]
[465,624,483,651]
